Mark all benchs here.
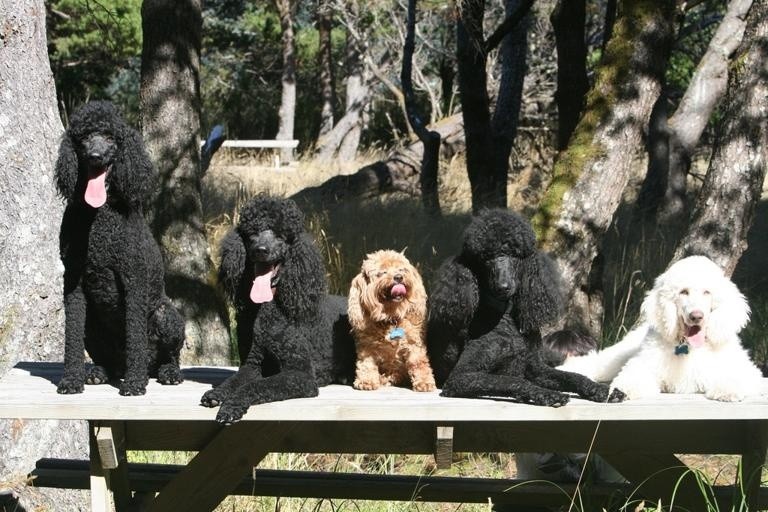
[199,138,300,169]
[0,361,768,512]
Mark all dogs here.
[197,191,355,427]
[50,100,188,395]
[427,205,627,409]
[554,252,768,404]
[345,243,441,395]
